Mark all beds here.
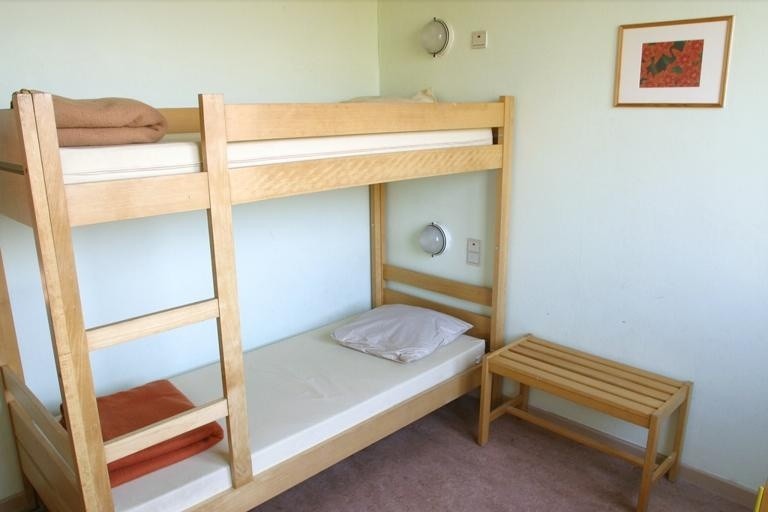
[0,85,517,310]
[0,267,511,510]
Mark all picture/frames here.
[611,13,734,110]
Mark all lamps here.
[418,222,452,259]
[419,16,456,58]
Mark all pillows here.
[329,301,474,362]
[344,87,438,103]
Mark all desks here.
[475,333,697,512]
